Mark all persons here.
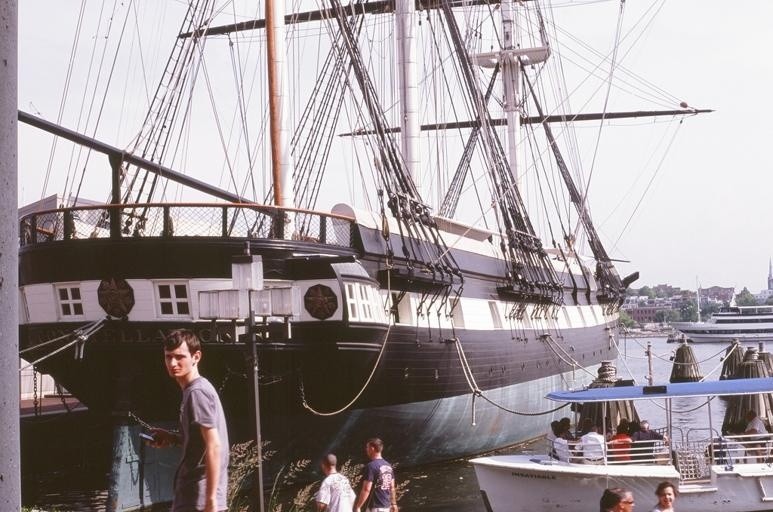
[352,435,400,512]
[315,453,359,511]
[740,410,769,463]
[599,488,626,511]
[548,416,669,462]
[142,327,229,511]
[621,491,635,512]
[647,480,681,511]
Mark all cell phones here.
[139,433,155,442]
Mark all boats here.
[469,378,773,512]
[670,305,773,343]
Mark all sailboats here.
[17,0,716,459]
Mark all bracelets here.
[390,503,397,505]
